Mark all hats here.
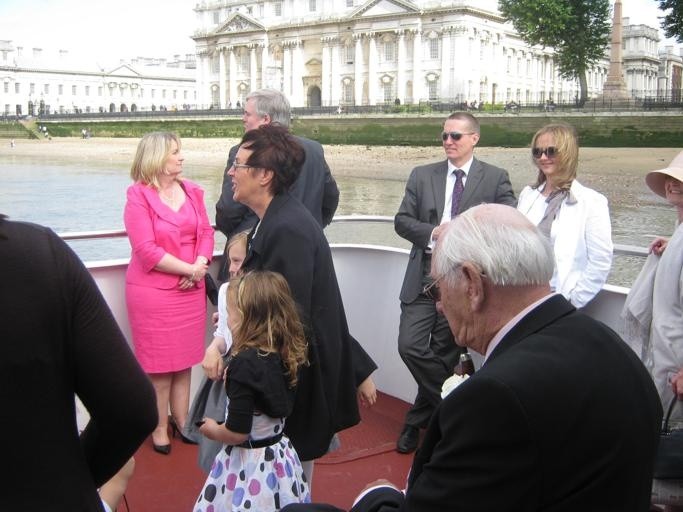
[645,150,683,199]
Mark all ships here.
[50,213,667,512]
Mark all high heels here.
[169,416,208,446]
[151,419,172,455]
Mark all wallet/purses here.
[202,273,220,307]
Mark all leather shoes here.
[397,422,422,454]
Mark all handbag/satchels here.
[653,391,683,483]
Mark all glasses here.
[422,260,483,303]
[232,160,268,170]
[531,146,560,159]
[441,131,474,141]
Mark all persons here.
[202,225,378,503]
[517,121,614,309]
[0,214,163,512]
[192,267,314,512]
[393,109,518,456]
[643,143,683,434]
[96,456,138,512]
[121,129,217,457]
[225,124,380,465]
[212,87,343,238]
[283,201,667,512]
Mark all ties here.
[450,169,466,219]
